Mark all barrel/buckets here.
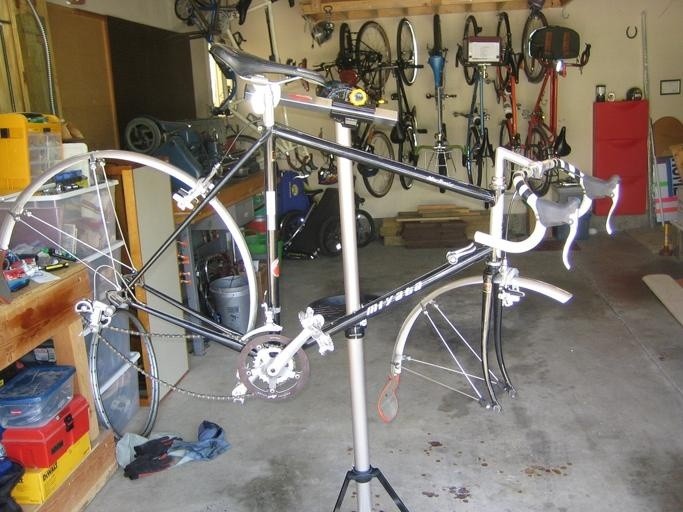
[209,275,250,336]
[277,169,311,214]
[241,228,284,267]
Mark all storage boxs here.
[1,394,90,468]
[0,365,76,428]
[0,180,120,262]
[82,300,131,389]
[75,240,125,301]
[94,351,141,436]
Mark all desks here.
[0,260,119,512]
[173,169,265,356]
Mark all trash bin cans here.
[551,183,591,241]
